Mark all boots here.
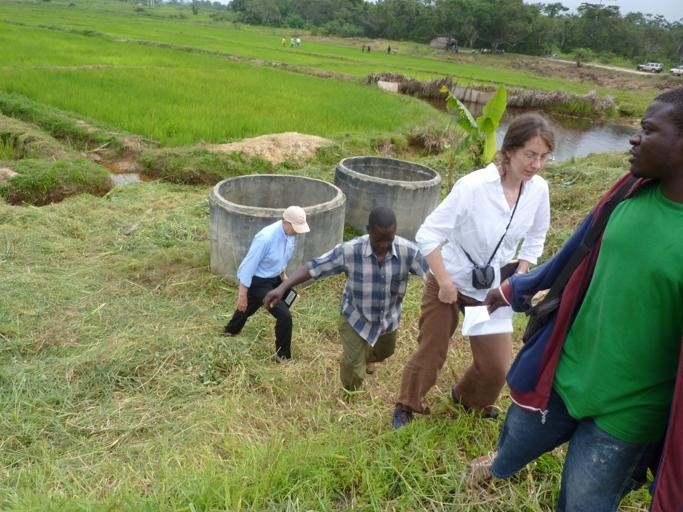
[366,363,375,375]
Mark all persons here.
[392,111,555,431]
[218,205,311,365]
[262,206,429,404]
[464,86,683,512]
[282,35,301,49]
[636,63,641,70]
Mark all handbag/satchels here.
[519,291,564,344]
[472,266,495,289]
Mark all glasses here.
[520,152,556,165]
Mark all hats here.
[282,205,310,234]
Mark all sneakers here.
[465,452,499,479]
[450,390,499,419]
[392,405,413,430]
